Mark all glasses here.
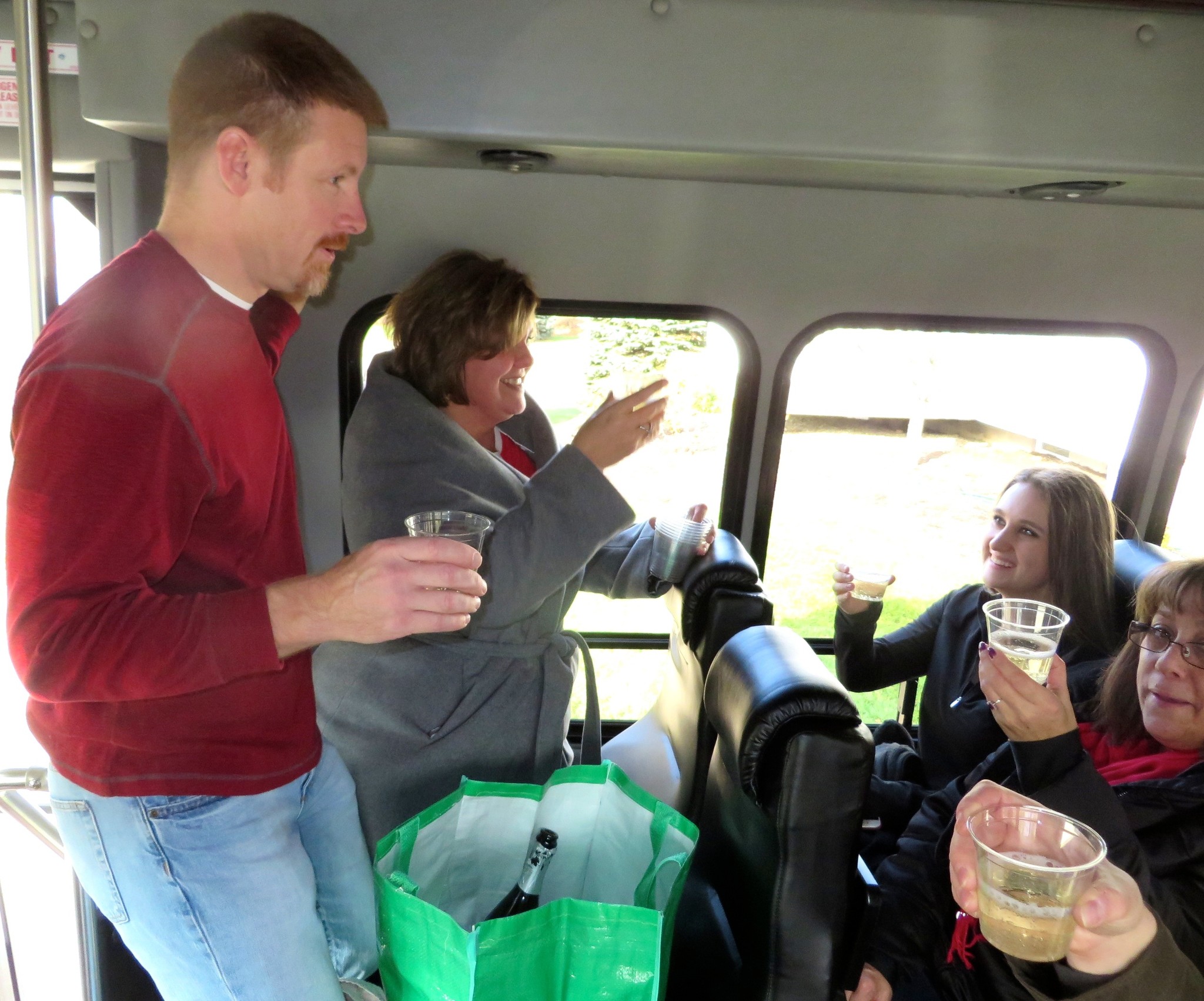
[1128,620,1204,670]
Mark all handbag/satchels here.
[372,762,699,1001]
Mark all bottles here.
[483,827,558,921]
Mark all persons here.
[832,462,1135,953]
[8,8,488,1001]
[309,248,715,882]
[833,558,1204,1001]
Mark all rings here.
[639,426,649,433]
[986,698,1002,710]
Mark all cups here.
[982,598,1072,685]
[648,505,712,583]
[612,373,663,412]
[966,805,1109,962]
[845,545,892,601]
[404,509,496,592]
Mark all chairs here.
[661,625,876,1001]
[600,528,775,810]
